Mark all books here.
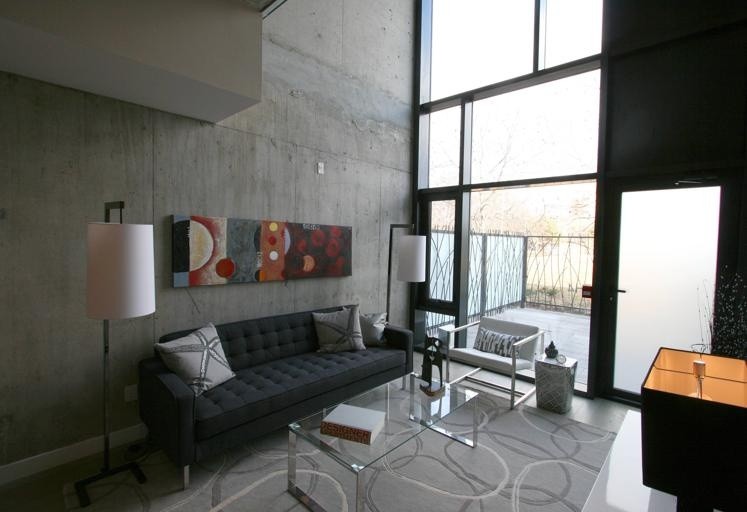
[320,404,386,444]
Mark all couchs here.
[138,306,414,491]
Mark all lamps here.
[387,222,426,322]
[641,346,747,512]
[74,201,157,512]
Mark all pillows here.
[473,327,527,359]
[153,322,236,397]
[312,303,389,354]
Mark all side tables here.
[535,353,577,415]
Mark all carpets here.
[0,372,617,512]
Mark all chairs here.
[446,316,545,409]
